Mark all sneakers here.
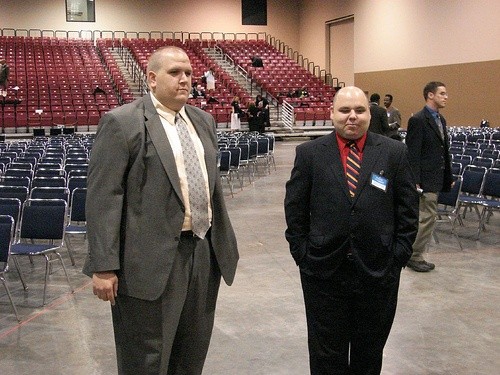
[407,261,429,272]
[421,261,434,269]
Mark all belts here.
[180,230,211,238]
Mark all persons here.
[287,86,309,98]
[190,71,214,99]
[230,94,271,134]
[367,93,396,136]
[251,56,263,67]
[92,86,106,96]
[404,81,457,273]
[80,45,239,375]
[382,94,402,138]
[284,86,420,375]
[0,59,10,88]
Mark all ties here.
[175,112,209,240]
[436,112,444,141]
[346,140,361,202]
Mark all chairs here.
[0,35,344,325]
[399,125,500,250]
